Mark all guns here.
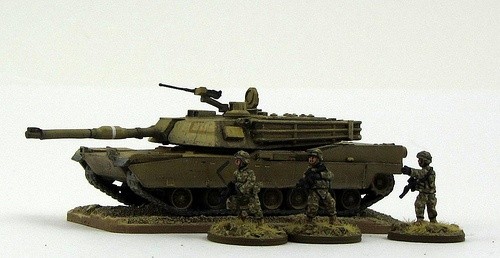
[291,164,325,197]
[221,181,238,202]
[159,81,224,101]
[398,178,420,199]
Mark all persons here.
[302,149,336,226]
[226,151,263,226]
[400,150,438,224]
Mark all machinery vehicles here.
[26,82,408,217]
